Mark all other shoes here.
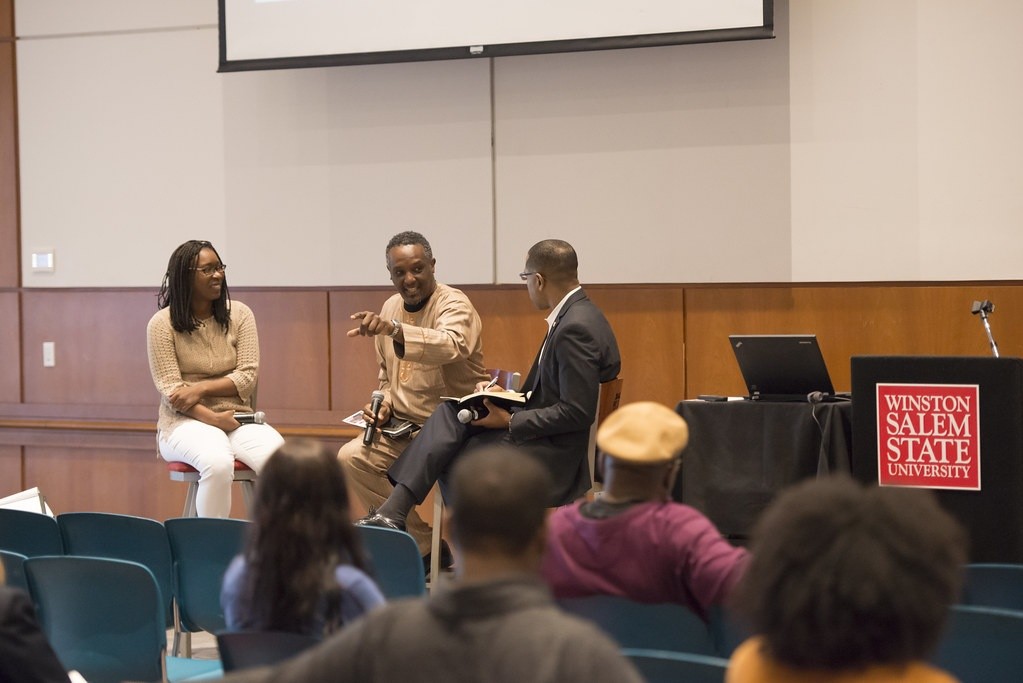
[421,546,454,579]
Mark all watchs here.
[388,319,402,337]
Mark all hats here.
[596,401,689,465]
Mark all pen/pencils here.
[484,376,499,391]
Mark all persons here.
[535,401,751,618]
[354,239,622,532]
[232,445,643,683]
[337,233,490,576]
[147,239,285,519]
[726,474,966,683]
[219,439,385,639]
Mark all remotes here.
[697,394,728,402]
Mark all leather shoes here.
[351,504,405,531]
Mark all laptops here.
[729,334,852,402]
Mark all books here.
[440,391,526,406]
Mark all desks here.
[675,392,852,538]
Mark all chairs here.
[0,367,1023,683]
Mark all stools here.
[169,461,258,519]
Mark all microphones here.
[363,390,384,445]
[457,408,514,423]
[234,411,266,424]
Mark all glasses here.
[187,264,226,276]
[519,273,545,284]
